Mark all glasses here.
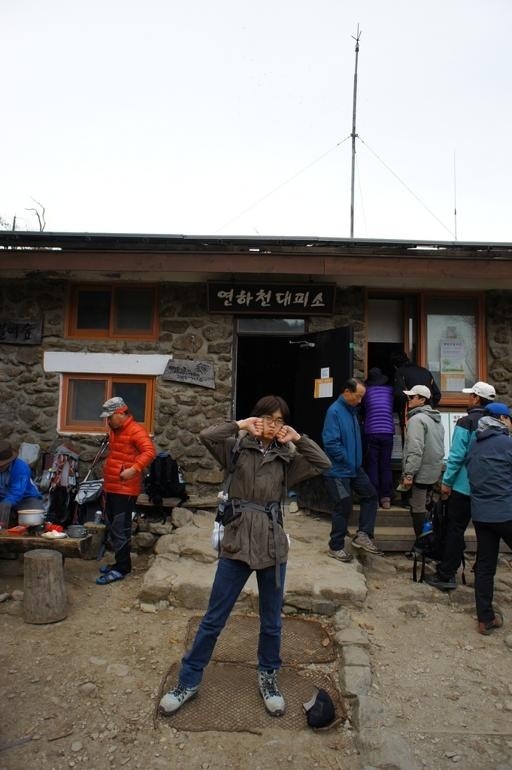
[265,417,284,427]
[409,395,415,400]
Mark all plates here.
[41,533,68,539]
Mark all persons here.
[1,442,44,526]
[393,351,441,505]
[360,363,395,508]
[402,384,444,560]
[422,381,496,589]
[158,394,332,715]
[97,397,155,587]
[463,402,512,636]
[322,377,384,561]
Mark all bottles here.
[94,507,103,525]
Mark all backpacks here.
[411,504,466,585]
[145,453,189,525]
[49,444,79,494]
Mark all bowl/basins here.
[18,509,45,527]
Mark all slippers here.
[97,564,124,585]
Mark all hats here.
[0,440,20,467]
[100,397,127,418]
[462,382,512,418]
[302,685,336,728]
[364,367,388,386]
[402,385,431,399]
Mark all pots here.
[67,525,99,538]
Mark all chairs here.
[401,499,469,588]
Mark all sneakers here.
[258,668,285,717]
[159,682,201,716]
[479,612,502,634]
[423,571,457,588]
[328,531,381,561]
